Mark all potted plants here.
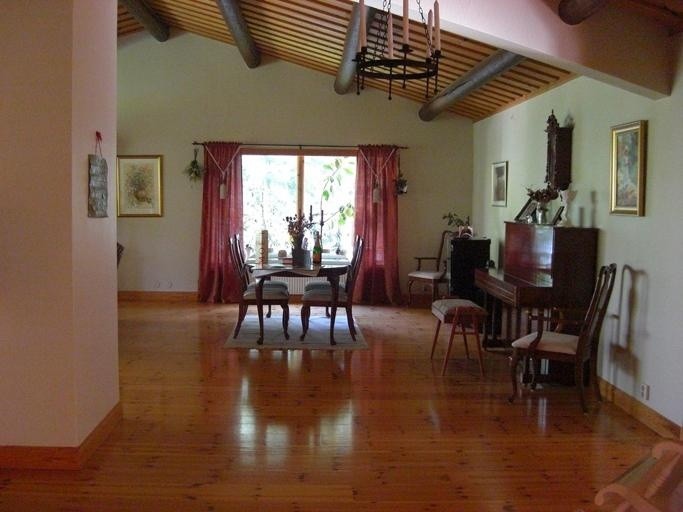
[441,208,474,238]
[522,180,560,225]
[182,146,206,193]
[392,153,410,195]
[312,157,357,264]
[284,206,315,269]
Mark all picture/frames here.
[490,160,509,208]
[513,197,540,223]
[116,153,164,219]
[607,119,648,217]
[551,206,565,226]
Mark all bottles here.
[219,179,227,200]
[312,231,322,267]
[372,183,380,204]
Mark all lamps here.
[350,0,445,102]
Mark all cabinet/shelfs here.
[449,238,491,306]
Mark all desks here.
[245,262,351,347]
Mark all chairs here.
[299,238,365,341]
[507,262,618,415]
[227,237,291,341]
[233,234,288,321]
[594,439,682,511]
[116,241,124,267]
[406,230,457,307]
[304,233,362,335]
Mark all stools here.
[429,298,490,379]
